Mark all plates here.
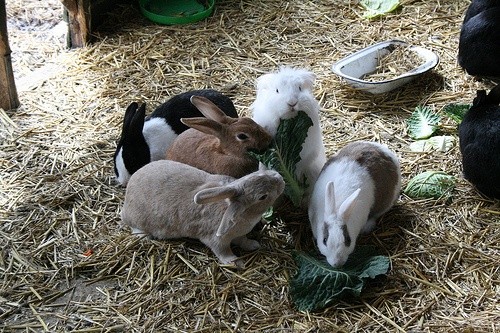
[140,0,214,24]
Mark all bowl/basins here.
[331,39,439,94]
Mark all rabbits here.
[110,66,402,273]
